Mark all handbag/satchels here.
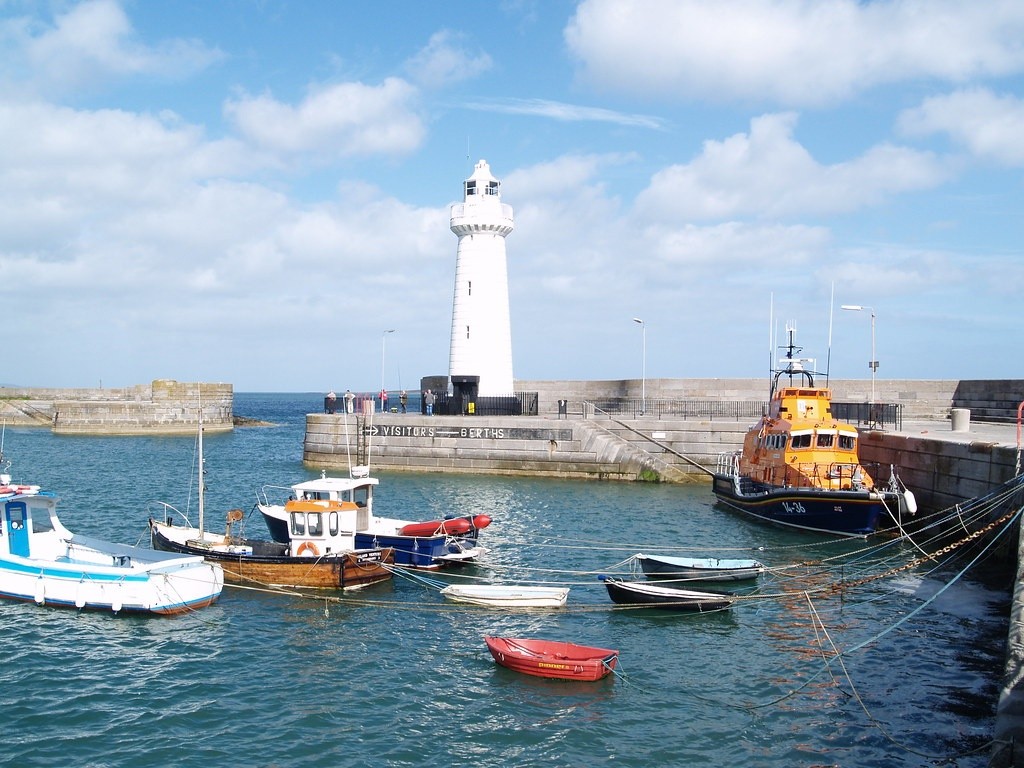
[895,475,917,514]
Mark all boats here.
[597,573,737,611]
[636,554,763,581]
[439,582,571,608]
[145,381,397,590]
[482,634,622,682]
[255,392,492,569]
[711,281,916,540]
[0,418,227,616]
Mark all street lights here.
[633,318,646,416]
[380,328,398,414]
[838,303,878,405]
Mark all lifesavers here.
[295,542,320,557]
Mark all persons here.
[327,390,336,414]
[345,390,355,413]
[399,390,407,414]
[378,389,388,414]
[424,389,434,416]
